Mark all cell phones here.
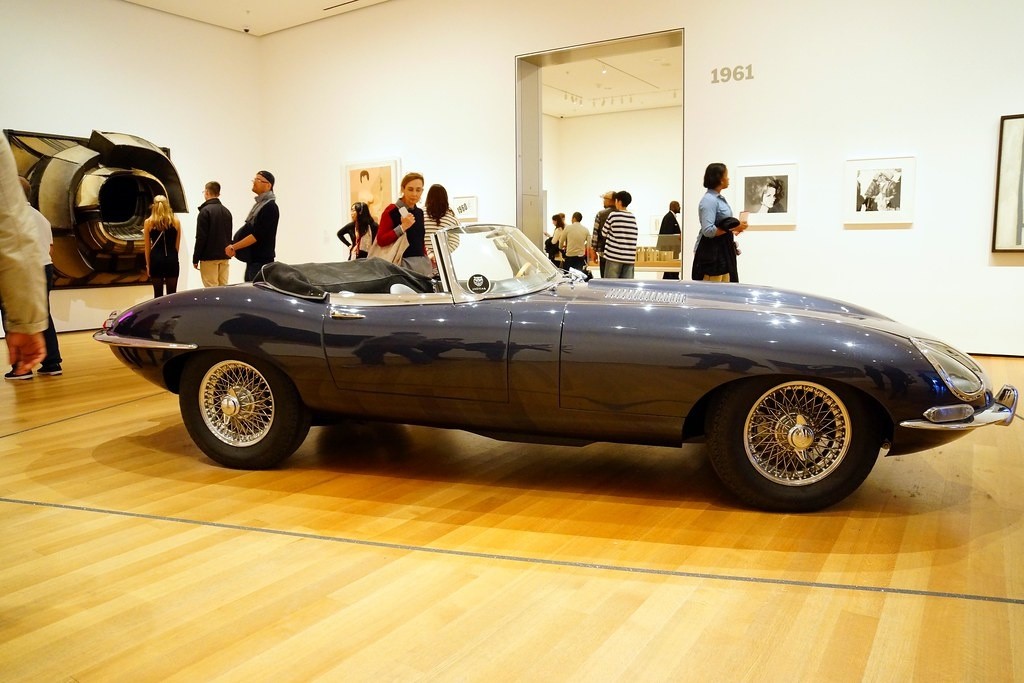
[399,207,409,218]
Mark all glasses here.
[252,179,269,183]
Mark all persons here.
[0,128,49,375]
[543,191,638,278]
[337,202,379,261]
[656,201,680,279]
[422,184,460,274]
[225,171,279,282]
[691,163,748,283]
[377,172,428,258]
[144,195,181,299]
[0,176,62,380]
[193,181,232,287]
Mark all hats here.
[258,171,275,186]
[601,191,616,199]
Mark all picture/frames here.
[340,156,402,257]
[842,156,915,224]
[990,114,1024,253]
[734,163,799,227]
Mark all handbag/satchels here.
[233,222,263,263]
[367,201,410,265]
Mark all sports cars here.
[93,225,1018,511]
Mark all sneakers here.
[5,369,34,380]
[37,363,62,375]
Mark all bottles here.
[636,246,658,262]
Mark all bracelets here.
[229,244,235,252]
